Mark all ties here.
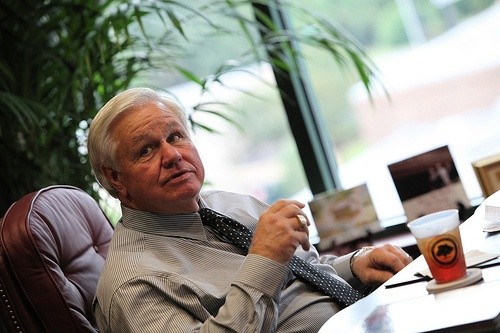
[199,208,365,307]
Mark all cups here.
[407,209,466,285]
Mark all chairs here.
[0,185,115,333]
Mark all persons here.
[86,88,415,333]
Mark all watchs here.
[349,245,375,273]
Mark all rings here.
[401,254,409,263]
[294,214,308,231]
